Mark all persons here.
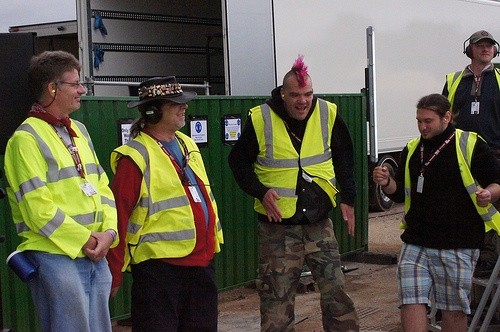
[442,30,500,262]
[3,50,120,332]
[227,56,360,332]
[104,76,224,332]
[373,94,500,332]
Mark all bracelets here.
[381,175,390,187]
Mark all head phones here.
[141,106,162,125]
[463,35,500,60]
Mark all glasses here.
[56,80,82,89]
[180,150,214,187]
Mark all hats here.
[126,76,197,109]
[470,30,495,44]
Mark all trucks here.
[75,0,500,212]
